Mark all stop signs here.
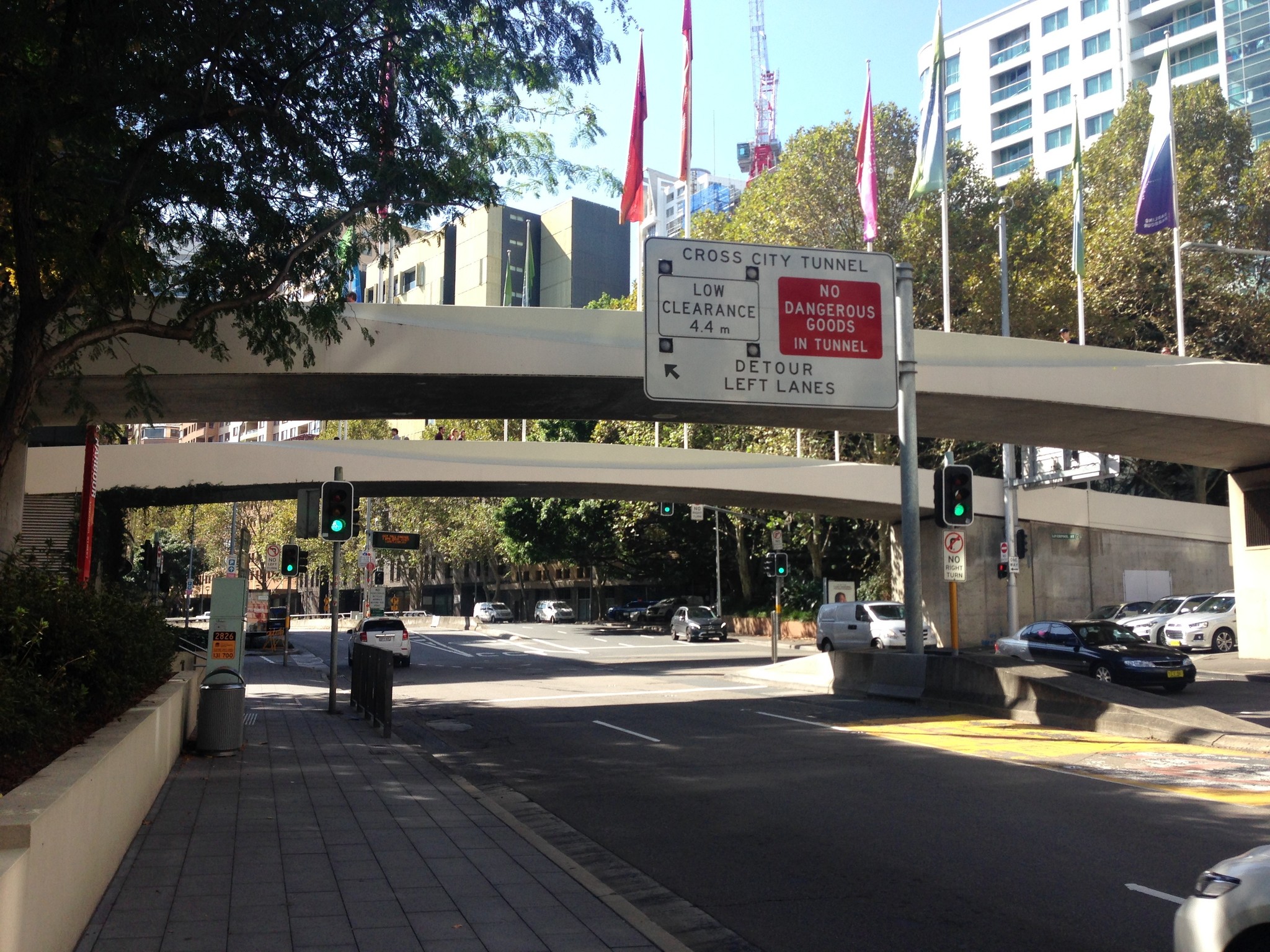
[1001,543,1007,553]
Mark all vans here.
[473,602,515,624]
[267,606,291,634]
[815,601,946,656]
[535,600,576,624]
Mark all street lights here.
[205,586,210,611]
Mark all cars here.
[195,611,210,622]
[605,596,704,622]
[994,619,1197,694]
[1170,840,1270,952]
[1082,600,1155,636]
[1164,589,1239,652]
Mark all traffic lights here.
[140,539,150,571]
[1016,529,1028,558]
[661,502,674,517]
[996,563,1007,578]
[375,571,384,586]
[281,544,308,577]
[320,481,361,543]
[764,553,787,577]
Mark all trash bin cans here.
[196,666,246,757]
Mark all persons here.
[435,426,445,440]
[457,429,465,440]
[1058,328,1077,344]
[346,291,357,303]
[835,592,846,602]
[1161,345,1171,355]
[449,428,458,441]
[391,428,399,440]
[334,437,340,440]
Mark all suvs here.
[347,615,411,667]
[670,606,728,643]
[1117,591,1218,647]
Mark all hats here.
[1058,328,1068,335]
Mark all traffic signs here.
[1009,557,1020,572]
[157,547,162,556]
[943,465,974,526]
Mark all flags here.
[376,25,394,219]
[619,34,647,225]
[1070,109,1086,277]
[1134,48,1177,234]
[855,69,879,242]
[333,225,362,303]
[908,5,945,201]
[679,0,693,182]
